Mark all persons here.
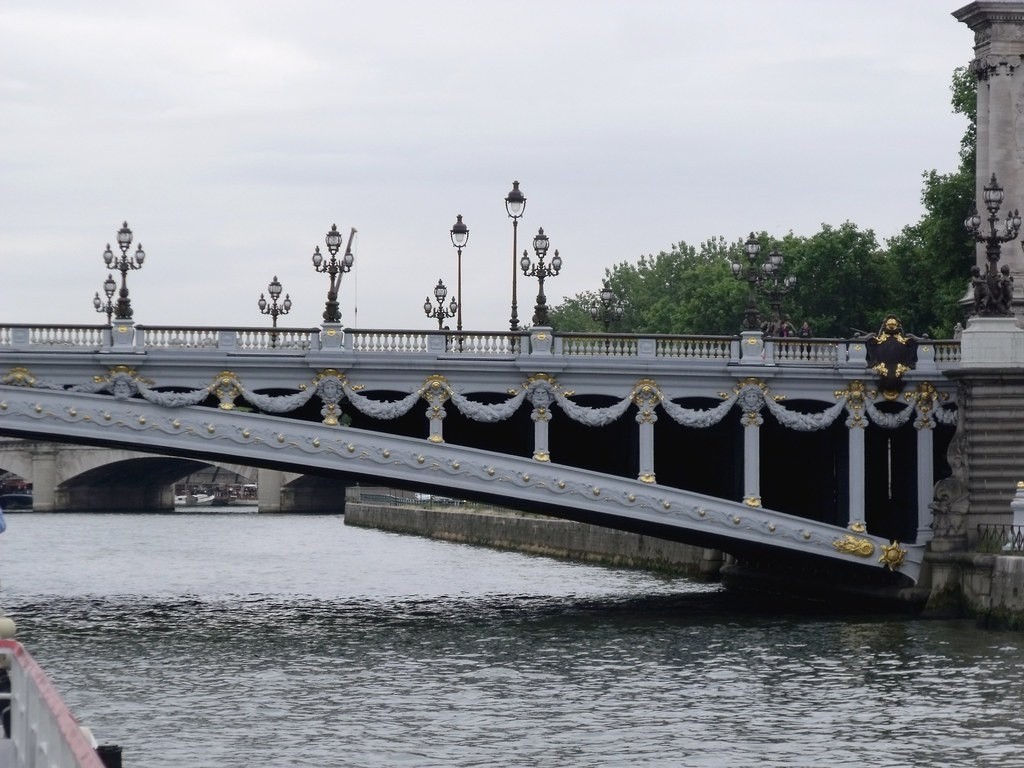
[776,320,812,361]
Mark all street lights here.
[92,273,115,332]
[963,172,1023,318]
[503,179,527,353]
[257,276,292,345]
[516,226,563,326]
[756,249,798,336]
[729,235,783,331]
[591,284,624,335]
[450,212,469,348]
[103,222,146,321]
[311,224,353,325]
[423,278,458,333]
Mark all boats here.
[175,480,260,506]
